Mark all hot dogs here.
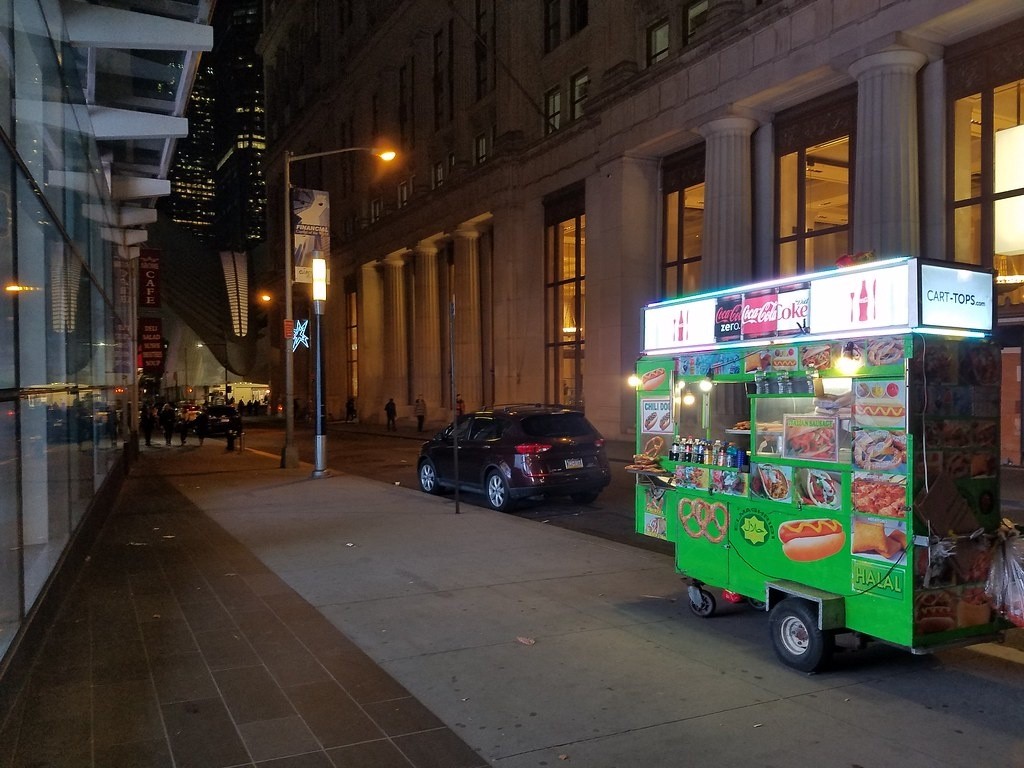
[645,412,658,430]
[659,412,670,431]
[778,518,845,561]
[771,357,796,369]
[641,368,666,390]
[912,606,955,633]
[787,425,835,457]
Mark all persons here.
[305,400,313,423]
[72,403,120,450]
[413,394,427,433]
[177,408,188,445]
[384,398,397,432]
[140,404,156,446]
[237,399,261,417]
[159,403,177,446]
[456,394,465,417]
[226,412,242,450]
[345,398,354,423]
[193,409,209,445]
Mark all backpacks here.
[353,409,357,418]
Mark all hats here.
[419,393,423,395]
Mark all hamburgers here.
[802,346,831,370]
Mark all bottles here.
[777,363,792,394]
[697,438,706,464]
[671,435,680,461]
[703,441,713,464]
[684,436,693,462]
[755,366,769,394]
[718,441,728,467]
[806,363,820,393]
[726,442,738,468]
[712,440,722,465]
[677,437,686,461]
[690,355,695,374]
[691,439,700,462]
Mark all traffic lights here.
[188,388,193,393]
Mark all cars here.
[175,403,201,422]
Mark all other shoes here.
[199,440,203,445]
[350,420,355,424]
[392,427,398,431]
[181,440,186,445]
[345,421,348,424]
[388,428,390,431]
[145,441,150,446]
[165,440,171,445]
[226,446,234,451]
[417,430,423,432]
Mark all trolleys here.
[625,258,1022,670]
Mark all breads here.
[852,520,909,559]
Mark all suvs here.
[198,404,243,437]
[417,399,614,509]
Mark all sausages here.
[851,404,905,417]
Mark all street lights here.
[197,343,228,405]
[310,232,333,482]
[279,148,398,469]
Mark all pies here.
[797,468,837,508]
[756,464,789,502]
